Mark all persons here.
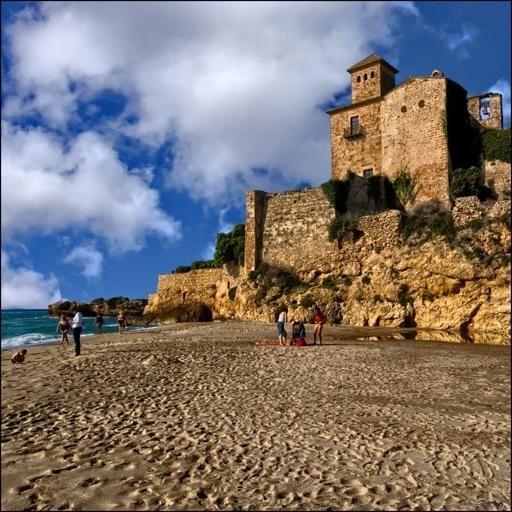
[277,305,324,346]
[11,349,27,364]
[117,311,126,335]
[95,313,104,335]
[56,306,83,356]
